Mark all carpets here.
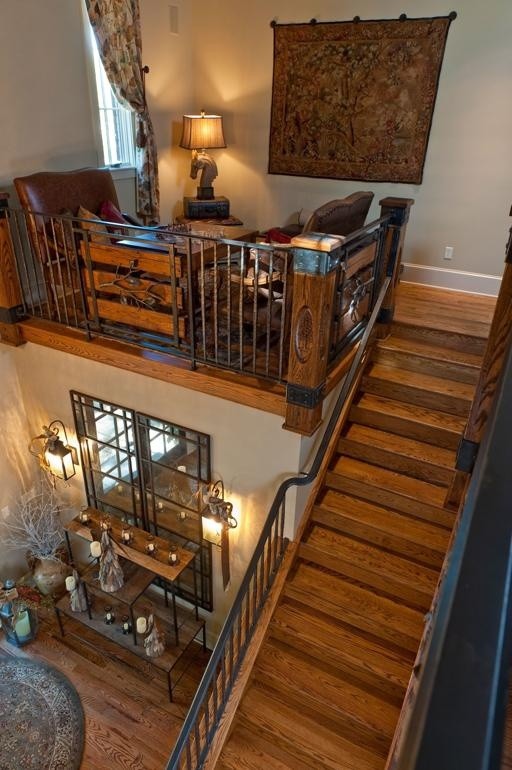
[0,655,86,770]
[78,311,280,369]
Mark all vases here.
[35,558,70,597]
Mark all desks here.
[176,213,244,226]
[52,505,210,702]
[114,219,261,351]
[97,483,210,551]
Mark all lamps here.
[42,418,80,482]
[198,479,238,553]
[178,107,231,200]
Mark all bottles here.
[121,525,133,544]
[80,505,90,524]
[99,512,112,531]
[104,605,115,624]
[167,545,181,566]
[120,615,131,634]
[144,534,158,555]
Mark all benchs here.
[8,162,151,323]
[207,189,379,371]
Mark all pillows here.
[246,221,304,305]
[49,199,132,258]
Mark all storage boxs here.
[183,194,231,220]
